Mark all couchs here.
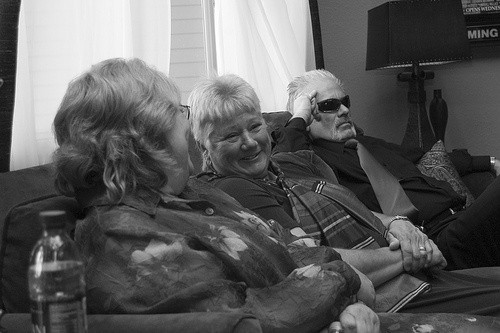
[0,111,500,333]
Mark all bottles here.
[429,89,448,142]
[447,149,472,178]
[27,211,88,333]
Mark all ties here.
[344,138,420,218]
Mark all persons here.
[271,69,500,271]
[186,72,500,317]
[50,57,500,333]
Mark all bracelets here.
[489,156,496,172]
[386,215,413,231]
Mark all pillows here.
[418,140,474,210]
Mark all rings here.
[418,247,426,251]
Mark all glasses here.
[180,105,190,120]
[317,95,351,112]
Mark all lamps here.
[366,0,472,151]
